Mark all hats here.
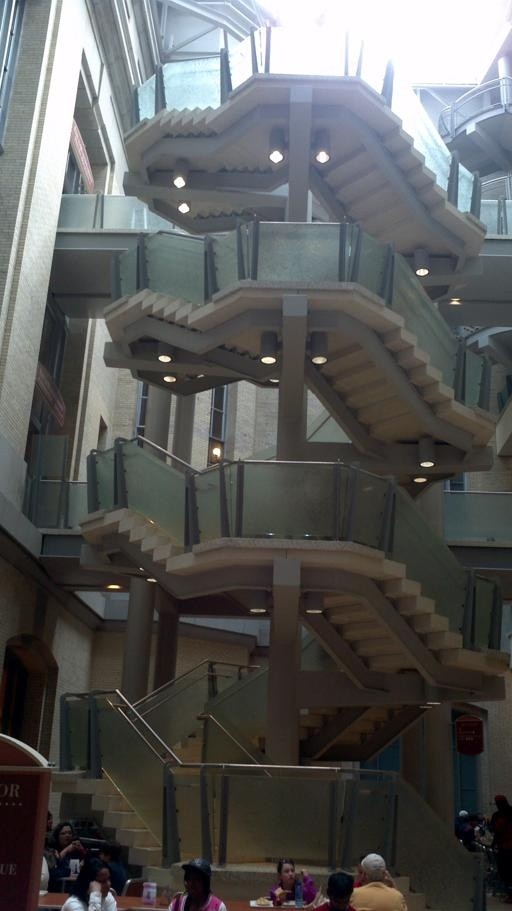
[182,858,211,876]
[361,852,386,874]
[328,872,354,896]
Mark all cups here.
[48,762,56,767]
[143,882,157,906]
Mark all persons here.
[61,856,119,911]
[454,794,511,900]
[351,856,408,911]
[310,871,357,911]
[352,858,397,888]
[168,858,228,911]
[268,857,317,907]
[38,810,128,898]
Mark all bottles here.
[295,880,303,908]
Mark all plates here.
[249,900,296,908]
